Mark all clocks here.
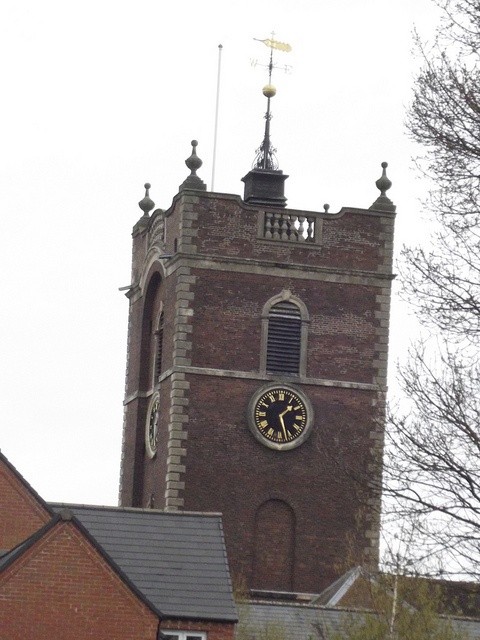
[146,391,160,459]
[249,384,315,451]
